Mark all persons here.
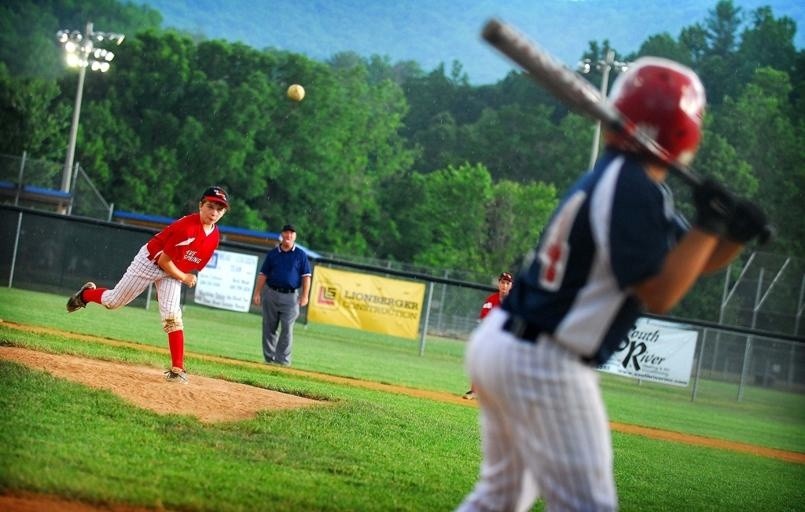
[252,225,313,369]
[64,184,232,386]
[451,47,772,510]
[462,272,515,400]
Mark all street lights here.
[575,50,626,173]
[55,23,126,213]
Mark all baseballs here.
[288,84,305,101]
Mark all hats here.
[499,271,513,283]
[204,185,229,210]
[282,224,295,233]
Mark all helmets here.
[602,57,707,164]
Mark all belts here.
[272,286,295,294]
[503,316,539,342]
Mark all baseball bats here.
[480,19,774,245]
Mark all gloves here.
[695,175,766,249]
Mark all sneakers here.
[463,390,475,399]
[66,282,96,312]
[168,367,187,383]
